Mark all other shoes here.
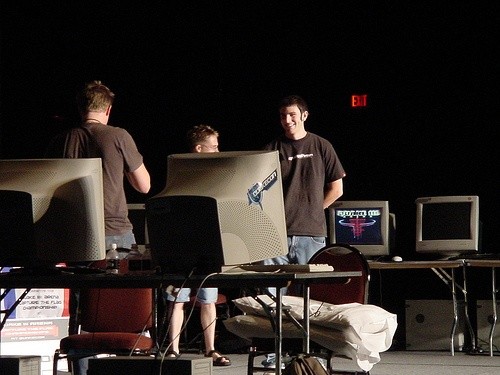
[264,362,285,375]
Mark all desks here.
[201,272,366,375]
[367,258,500,356]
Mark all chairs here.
[52,259,152,375]
[245,245,371,375]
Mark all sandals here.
[165,351,182,357]
[204,350,231,366]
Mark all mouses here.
[392,256,403,262]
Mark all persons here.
[163,124,232,367]
[62,79,150,259]
[252,94,346,302]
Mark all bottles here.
[105,244,119,274]
[141,244,156,276]
[128,244,142,276]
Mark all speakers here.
[476,300,500,355]
[404,299,467,353]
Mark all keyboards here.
[241,264,335,273]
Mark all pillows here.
[222,295,399,372]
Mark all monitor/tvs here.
[147,152,289,276]
[326,201,391,263]
[0,157,108,273]
[415,196,479,262]
